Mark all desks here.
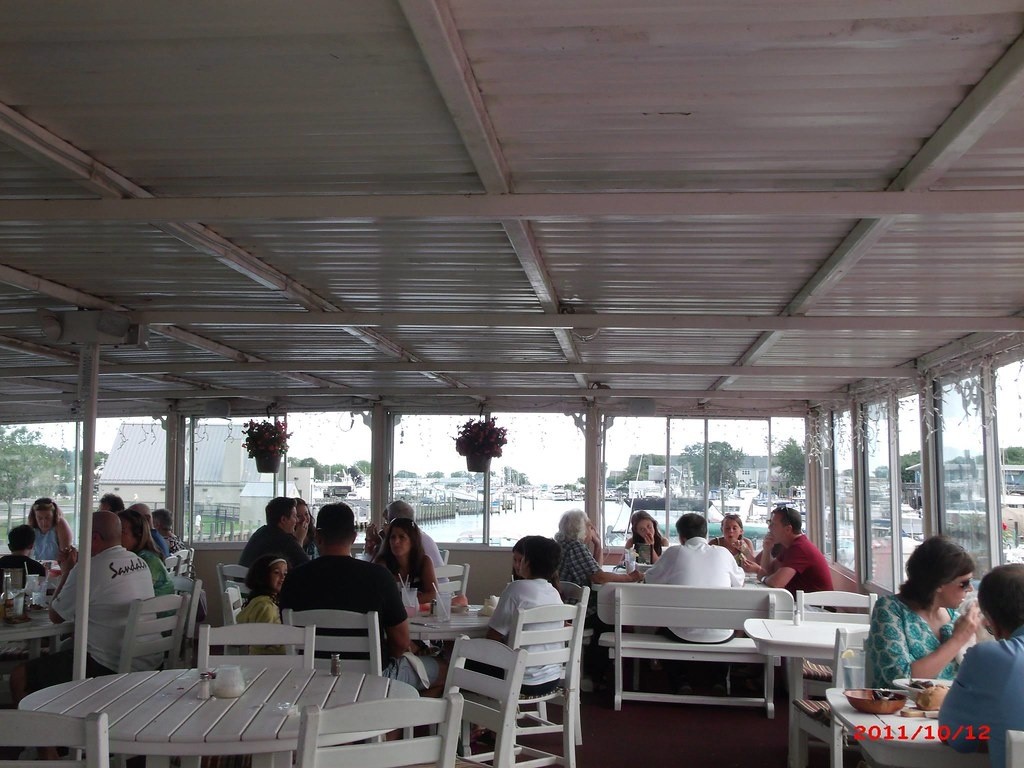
[743,618,871,768]
[18,665,419,768]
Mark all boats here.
[624,476,714,512]
[739,478,923,555]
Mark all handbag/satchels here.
[186,564,208,622]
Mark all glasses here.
[777,506,789,513]
[390,518,415,530]
[950,577,974,589]
[766,520,771,524]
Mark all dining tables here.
[385,603,492,662]
[592,565,772,592]
[826,688,989,768]
[0,608,75,662]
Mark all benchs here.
[596,583,793,719]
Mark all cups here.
[438,591,451,622]
[959,598,978,619]
[402,587,418,618]
[23,575,39,606]
[638,543,651,564]
[624,549,636,574]
[397,582,410,596]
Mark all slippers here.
[731,666,762,678]
[742,677,762,693]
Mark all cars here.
[551,490,566,501]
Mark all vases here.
[255,456,281,473]
[972,553,1006,580]
[467,456,490,472]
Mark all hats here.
[388,501,414,520]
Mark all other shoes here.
[649,659,664,670]
[673,684,693,695]
[712,682,727,696]
[471,725,497,748]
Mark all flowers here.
[450,417,507,460]
[242,419,294,458]
[947,513,1013,550]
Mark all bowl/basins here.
[638,565,652,572]
[842,690,907,713]
[893,678,953,715]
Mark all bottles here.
[331,653,340,676]
[0,572,48,624]
[45,569,63,596]
[212,666,246,698]
[197,672,212,702]
[431,599,437,615]
[793,609,801,626]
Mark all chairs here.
[0,544,878,768]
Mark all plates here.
[450,606,468,614]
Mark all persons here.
[237,497,835,760]
[866,535,982,691]
[0,492,181,760]
[938,563,1024,768]
